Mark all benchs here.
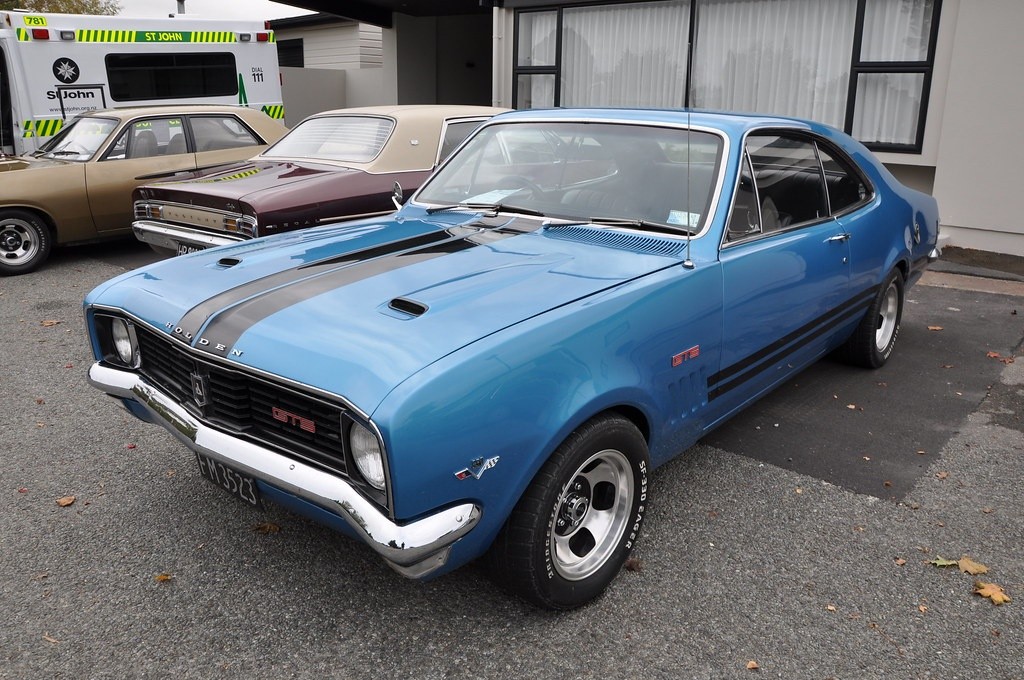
[639,163,845,219]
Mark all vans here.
[0,11,285,159]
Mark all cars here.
[83,110,944,613]
[0,101,292,276]
[131,105,619,257]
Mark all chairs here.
[130,131,158,159]
[164,134,188,155]
[561,189,638,215]
